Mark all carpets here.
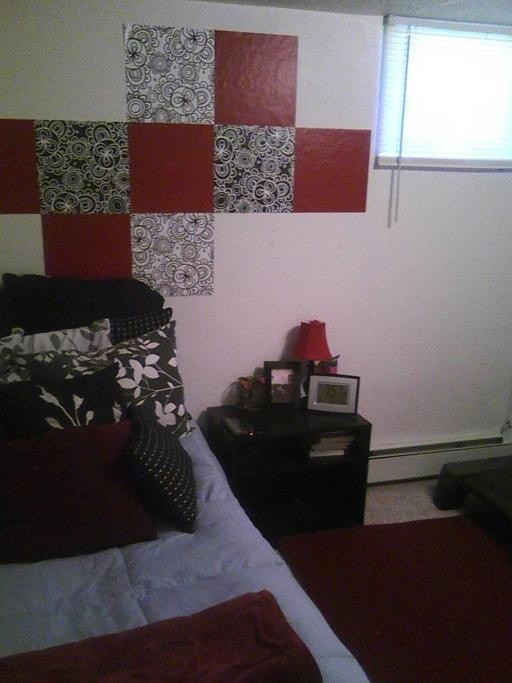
[276,515,511,682]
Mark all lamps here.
[290,319,332,396]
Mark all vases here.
[232,408,273,433]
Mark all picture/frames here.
[262,361,302,412]
[306,372,361,416]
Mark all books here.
[300,429,354,459]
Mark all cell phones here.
[222,417,256,437]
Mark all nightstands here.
[205,406,373,536]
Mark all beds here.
[1,272,369,683]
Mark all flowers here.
[228,366,274,410]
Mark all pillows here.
[0,306,198,564]
